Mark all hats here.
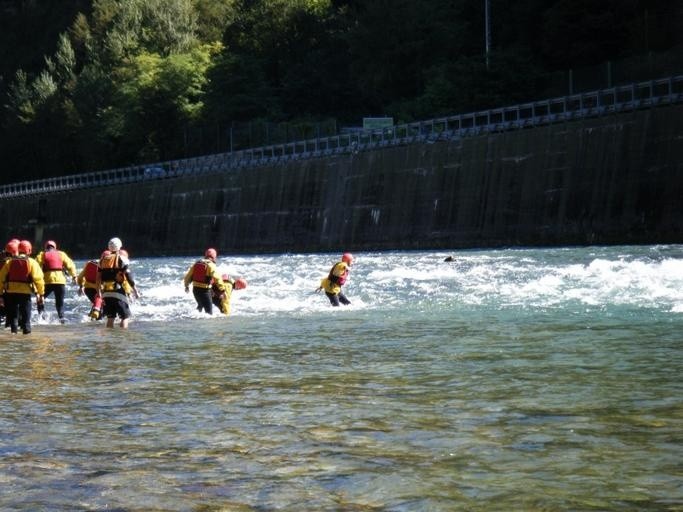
[6,237,33,255]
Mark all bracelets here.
[126,292,130,296]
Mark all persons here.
[95,237,140,328]
[77,259,106,321]
[118,250,133,304]
[0,238,44,334]
[34,240,77,325]
[182,248,227,316]
[314,253,352,307]
[196,273,247,315]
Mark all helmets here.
[109,237,123,253]
[342,252,353,262]
[44,239,57,249]
[235,277,247,288]
[205,247,217,258]
